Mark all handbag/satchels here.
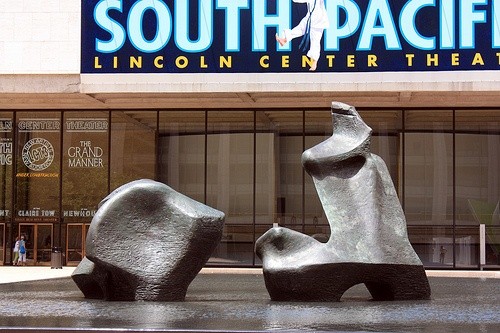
[20,246,25,254]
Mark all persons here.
[12,237,20,265]
[17,236,27,266]
[274,0,329,72]
[439,245,448,266]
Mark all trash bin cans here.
[51,246,62,269]
